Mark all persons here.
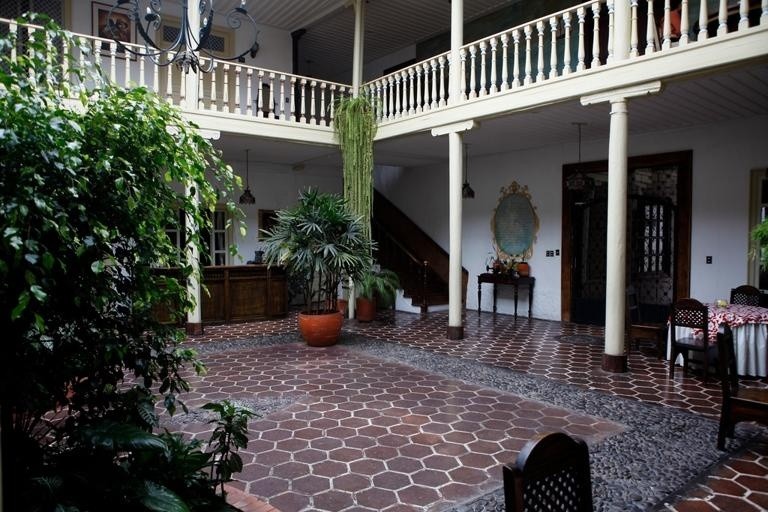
[99,16,129,43]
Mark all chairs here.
[502,431,595,512]
[717,322,768,450]
[669,298,716,387]
[625,282,668,357]
[730,285,766,307]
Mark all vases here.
[325,298,348,317]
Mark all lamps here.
[462,143,474,199]
[107,0,261,74]
[239,149,256,205]
[565,122,592,191]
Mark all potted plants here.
[255,183,378,347]
[355,263,400,324]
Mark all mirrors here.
[490,181,540,261]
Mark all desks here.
[478,273,535,322]
[667,302,768,378]
[694,0,762,38]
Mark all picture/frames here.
[91,0,138,62]
